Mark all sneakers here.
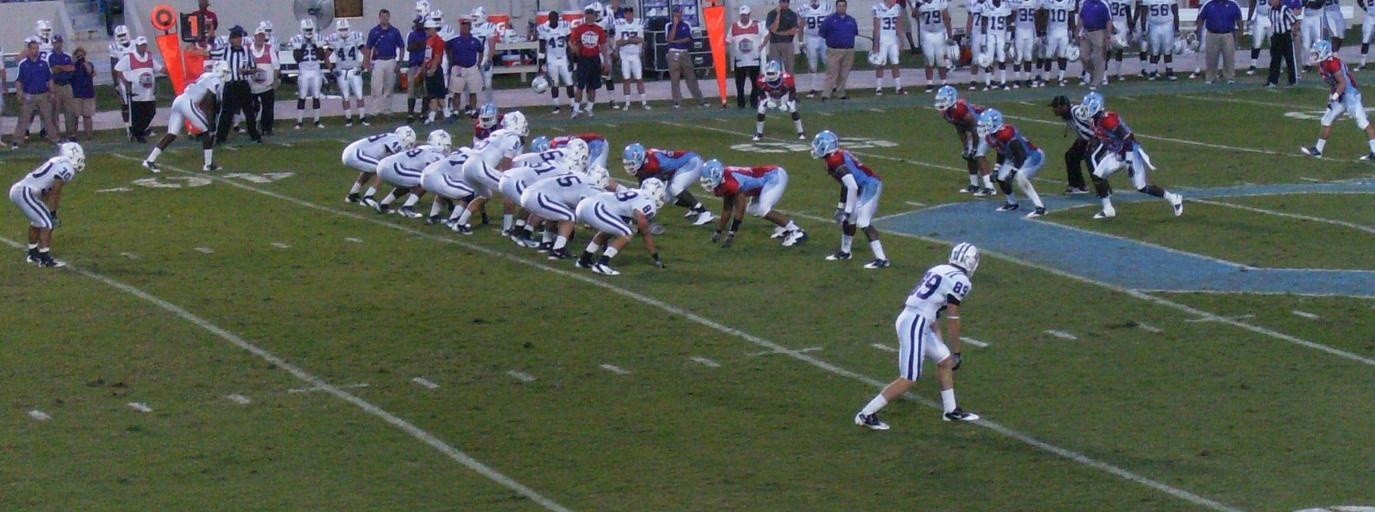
[854,412,890,430]
[959,185,997,197]
[502,226,621,276]
[1093,208,1115,219]
[1190,71,1235,84]
[926,84,934,94]
[863,258,890,269]
[554,102,652,118]
[770,227,807,247]
[142,160,160,173]
[996,202,1048,218]
[969,75,1066,91]
[26,252,66,268]
[943,407,979,422]
[1065,185,1088,195]
[684,208,715,225]
[875,88,883,96]
[806,89,847,101]
[1173,193,1184,217]
[346,117,369,128]
[1137,69,1178,81]
[895,88,907,95]
[1246,65,1256,75]
[1360,153,1375,160]
[826,250,852,261]
[1302,147,1321,159]
[406,110,478,127]
[1079,75,1125,90]
[203,165,222,171]
[345,193,473,235]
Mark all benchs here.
[273,44,411,83]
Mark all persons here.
[1,1,1370,275]
[855,243,979,433]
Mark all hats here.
[135,37,147,45]
[739,6,750,15]
[1047,96,1069,106]
[229,21,271,36]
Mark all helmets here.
[949,243,981,277]
[60,142,85,172]
[700,159,724,192]
[1077,92,1104,121]
[337,20,349,41]
[948,39,1081,68]
[1172,33,1199,55]
[395,125,452,150]
[114,25,129,44]
[641,177,665,209]
[532,76,548,94]
[300,19,314,38]
[412,0,484,28]
[1309,40,1332,63]
[213,60,232,83]
[867,50,884,67]
[623,143,645,176]
[25,20,62,48]
[976,108,1003,137]
[478,102,609,188]
[809,129,836,159]
[1111,36,1129,50]
[935,86,958,111]
[590,3,604,21]
[765,60,781,83]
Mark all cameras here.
[77,58,85,63]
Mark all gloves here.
[952,353,962,371]
[1125,160,1134,177]
[989,169,1016,183]
